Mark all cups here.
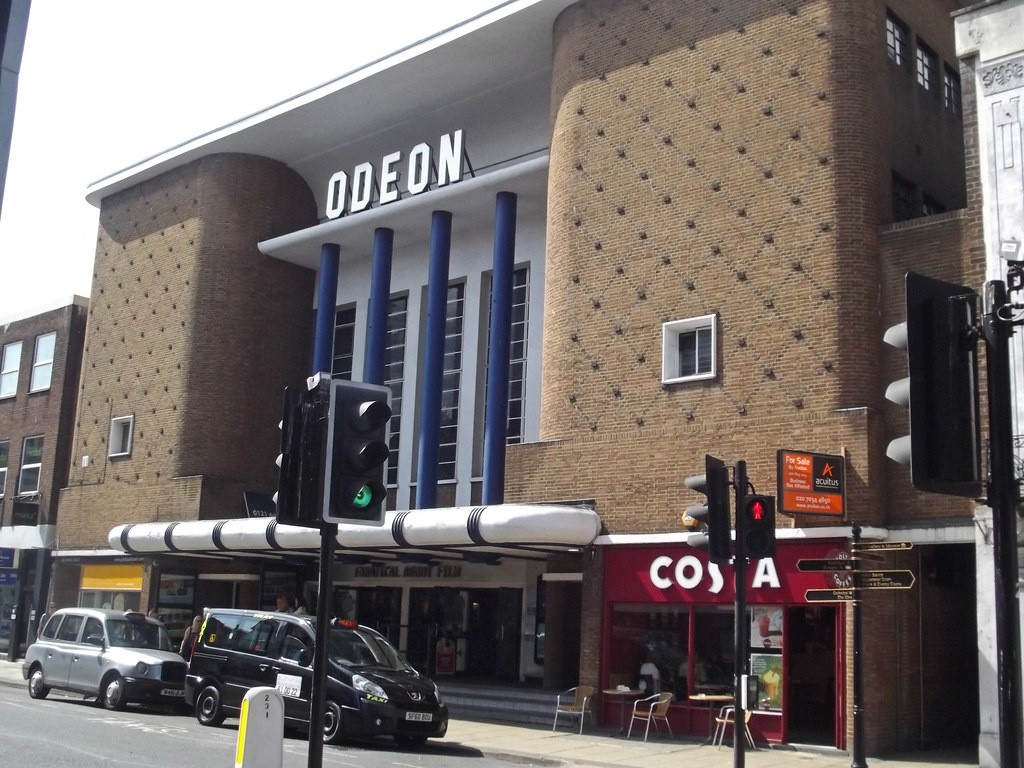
[763,671,780,700]
[758,616,770,637]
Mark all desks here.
[694,683,720,690]
[603,689,644,738]
[689,695,734,746]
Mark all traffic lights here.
[737,490,777,560]
[682,452,729,565]
[884,271,982,499]
[322,377,393,529]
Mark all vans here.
[183,606,450,747]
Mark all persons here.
[179,616,202,662]
[641,649,706,700]
[788,640,822,694]
[142,608,158,645]
[36,606,56,642]
[274,594,309,615]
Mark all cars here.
[21,606,189,712]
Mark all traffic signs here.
[859,570,917,590]
[857,540,912,552]
[803,588,858,603]
[855,551,885,562]
[795,559,858,571]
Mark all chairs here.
[712,705,756,749]
[552,685,594,735]
[626,692,674,742]
